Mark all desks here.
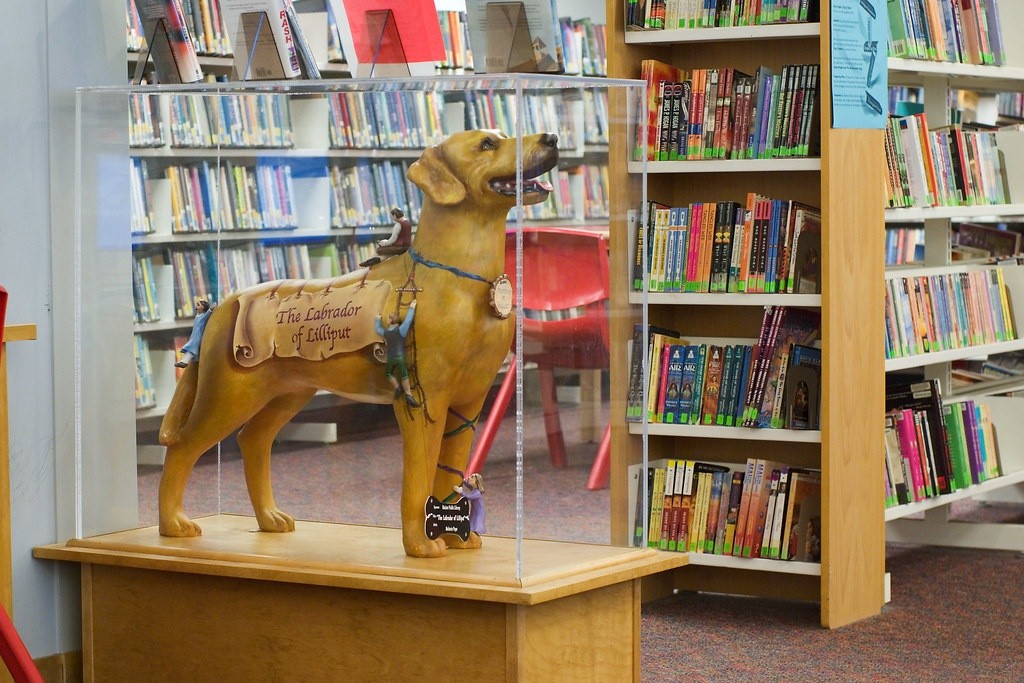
[32,514,689,683]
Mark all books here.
[632,60,821,162]
[884,228,1015,360]
[126,0,609,411]
[885,362,1023,510]
[885,84,1024,209]
[626,306,821,430]
[634,458,821,564]
[627,0,809,30]
[631,192,821,294]
[887,0,1006,66]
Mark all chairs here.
[457,224,612,491]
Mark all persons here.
[377,208,412,255]
[175,300,216,367]
[453,473,487,535]
[374,299,417,406]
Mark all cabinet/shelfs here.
[125,77,607,466]
[605,0,1024,630]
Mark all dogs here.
[155,127,561,558]
